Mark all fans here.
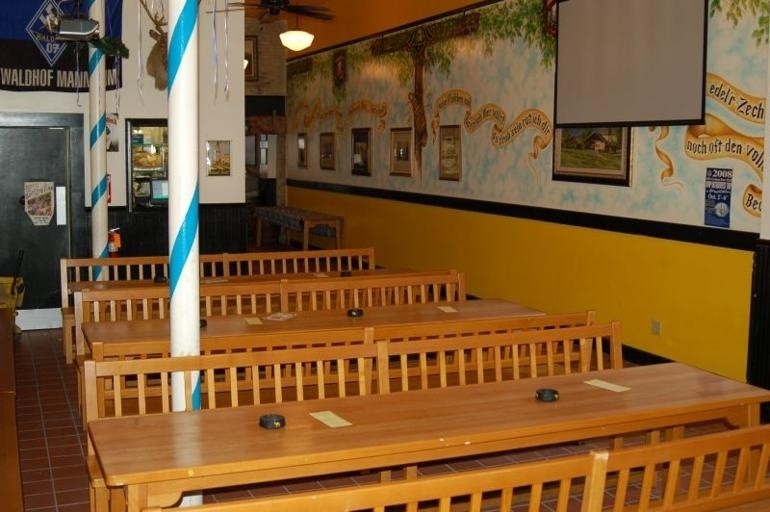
[205,0,336,22]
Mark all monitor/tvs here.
[150,177,168,205]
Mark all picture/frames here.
[296,124,461,182]
[244,34,260,80]
[550,127,632,188]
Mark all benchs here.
[137,422,770,512]
[59,246,624,456]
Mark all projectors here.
[55,17,99,42]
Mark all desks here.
[254,206,342,251]
[85,362,770,512]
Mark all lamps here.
[277,2,316,53]
